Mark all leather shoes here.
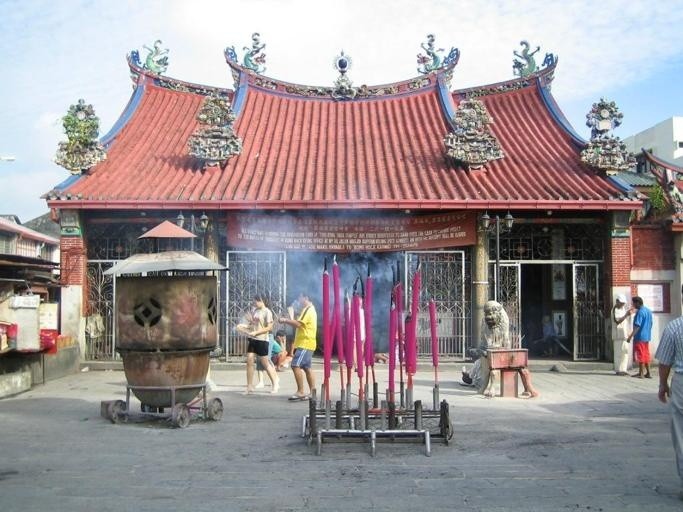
[616,371,627,375]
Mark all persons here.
[243,294,279,396]
[269,329,289,372]
[654,311,683,479]
[534,312,555,357]
[458,349,487,389]
[626,296,651,378]
[610,295,634,375]
[278,290,317,401]
[556,315,564,336]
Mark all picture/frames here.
[551,227,569,340]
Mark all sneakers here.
[246,384,264,393]
[632,373,650,378]
[271,378,280,394]
[288,393,313,401]
